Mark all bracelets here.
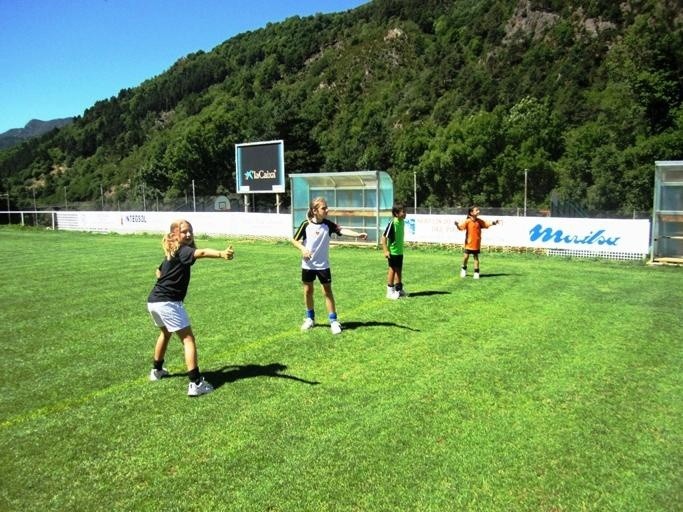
[491,221,496,225]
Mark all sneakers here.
[386,286,407,300]
[150,367,169,381]
[301,317,315,330]
[187,376,214,397]
[473,273,480,280]
[330,321,342,335]
[461,269,467,278]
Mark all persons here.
[144,218,235,398]
[453,203,504,280]
[379,203,409,300]
[290,196,367,337]
